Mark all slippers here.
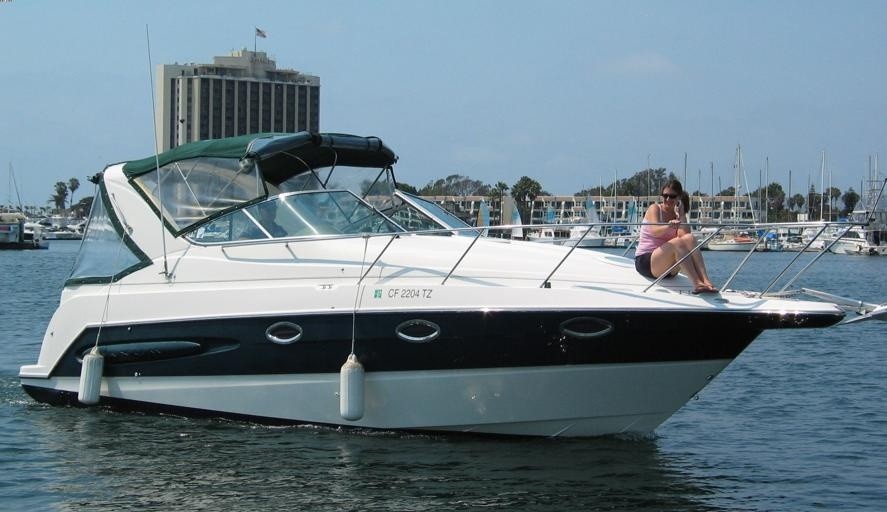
[691,285,711,293]
[710,284,718,292]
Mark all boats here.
[17,131,845,440]
[0,213,89,249]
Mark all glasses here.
[662,194,679,199]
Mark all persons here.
[237,200,288,239]
[634,179,718,294]
